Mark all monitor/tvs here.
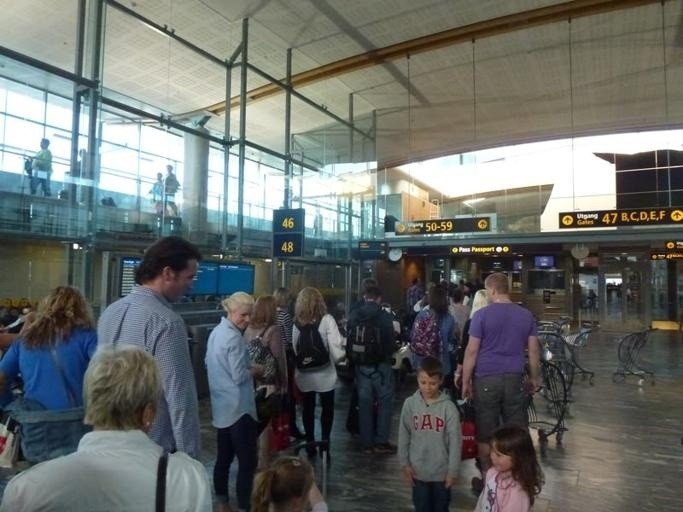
[119,256,146,298]
[184,260,219,296]
[218,262,255,296]
[533,254,555,268]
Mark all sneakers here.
[290,423,332,454]
[372,442,399,455]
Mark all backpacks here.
[410,306,444,358]
[291,313,331,374]
[344,309,387,368]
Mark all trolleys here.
[610,324,659,383]
[523,315,602,449]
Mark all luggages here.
[346,385,382,439]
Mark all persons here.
[158,164,178,216]
[312,209,324,238]
[76,147,89,202]
[147,172,166,214]
[0,343,213,510]
[97,233,201,460]
[0,283,97,459]
[586,288,597,316]
[28,137,53,199]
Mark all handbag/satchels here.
[455,394,479,461]
[0,414,21,472]
[269,410,291,451]
[245,322,282,384]
